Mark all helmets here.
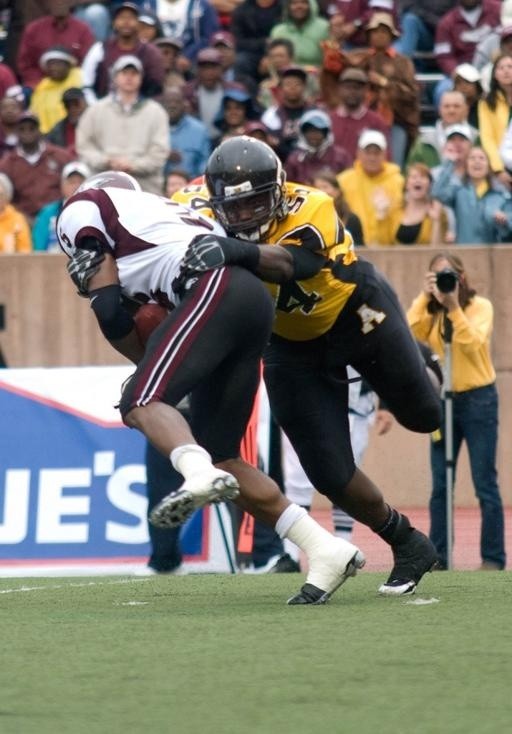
[205,132,288,243]
[71,170,142,195]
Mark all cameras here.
[436,271,456,294]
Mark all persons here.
[167,135,446,597]
[280,365,395,571]
[403,252,506,571]
[54,167,365,605]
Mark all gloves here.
[179,233,261,276]
[65,245,108,293]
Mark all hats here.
[4,0,403,150]
[451,61,483,82]
[443,124,473,142]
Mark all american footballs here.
[133,304,168,345]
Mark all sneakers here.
[289,537,366,603]
[275,553,503,571]
[415,341,444,388]
[147,469,240,529]
[377,528,439,595]
